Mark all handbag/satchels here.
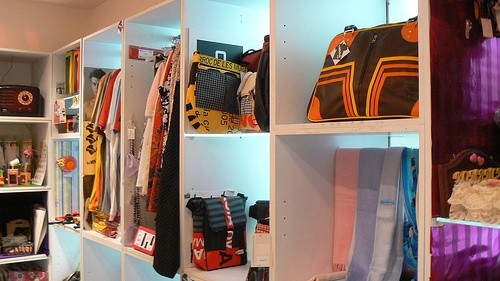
[185,37,269,133]
[307,18,420,120]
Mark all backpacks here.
[186,194,247,271]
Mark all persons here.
[84,69,106,121]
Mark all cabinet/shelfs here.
[0,0,500,281]
